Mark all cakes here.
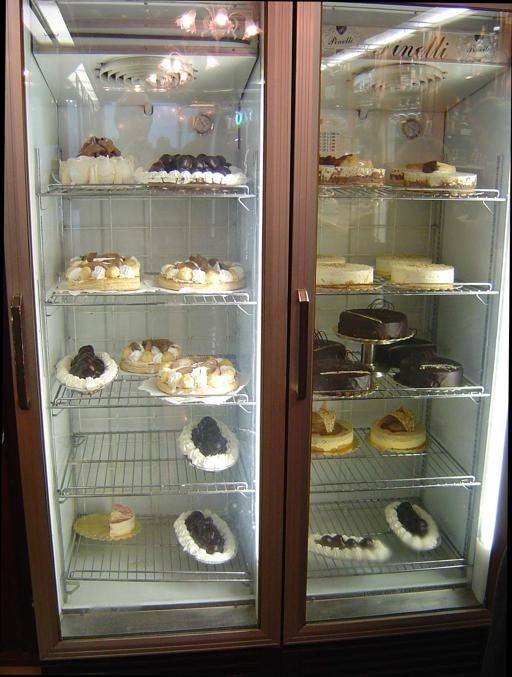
[176,416,241,471]
[318,153,385,187]
[158,254,247,293]
[317,260,349,282]
[109,501,134,536]
[311,401,354,457]
[386,499,441,552]
[121,336,182,375]
[338,308,411,338]
[56,345,116,391]
[390,263,456,289]
[395,354,467,390]
[317,264,377,289]
[314,361,377,396]
[374,255,437,282]
[138,153,248,188]
[389,161,480,196]
[308,532,391,563]
[157,355,239,399]
[377,339,436,370]
[55,133,135,189]
[172,510,237,565]
[371,405,429,454]
[314,336,346,364]
[58,252,139,292]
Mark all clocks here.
[194,112,213,135]
[403,119,421,139]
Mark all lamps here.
[176,4,261,45]
[160,52,219,82]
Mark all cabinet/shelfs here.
[3,1,295,662]
[284,2,512,644]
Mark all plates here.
[312,430,362,457]
[70,512,145,542]
[136,373,251,408]
[367,435,428,455]
